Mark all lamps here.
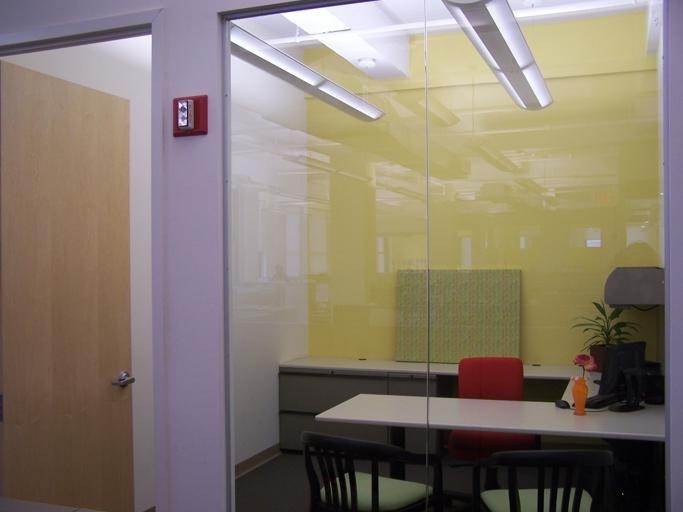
[438,0,552,112]
[229,21,385,124]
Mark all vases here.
[569,374,588,417]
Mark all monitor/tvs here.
[599,340,646,412]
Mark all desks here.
[278,353,666,512]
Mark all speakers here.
[645,375,664,405]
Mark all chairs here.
[474,448,629,512]
[445,356,540,490]
[299,429,445,512]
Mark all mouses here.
[554,400,569,408]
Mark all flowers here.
[572,353,594,377]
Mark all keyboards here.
[572,393,625,409]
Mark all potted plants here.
[568,297,641,372]
